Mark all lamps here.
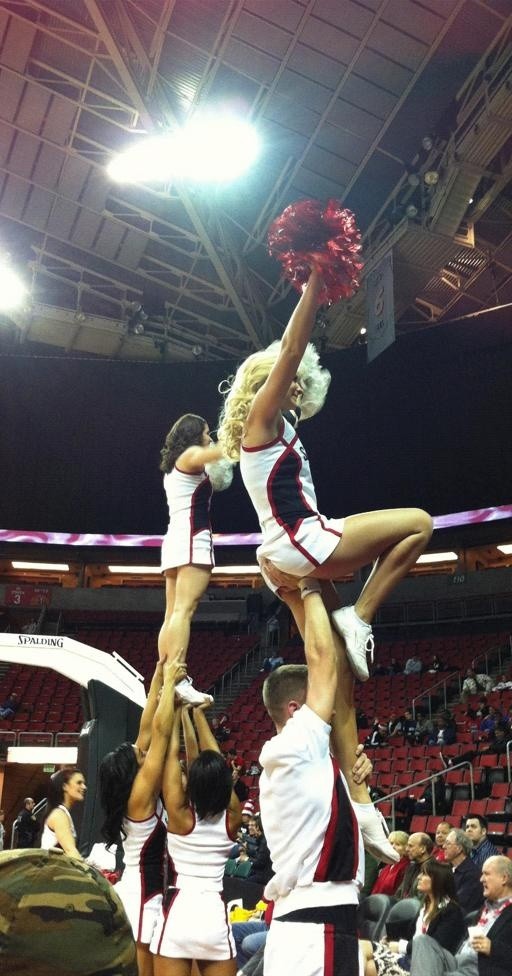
[192,346,201,357]
[121,300,147,335]
[73,312,85,322]
[405,129,449,218]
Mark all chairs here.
[0,606,166,747]
[179,620,305,812]
[355,633,512,859]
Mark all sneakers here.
[330,604,375,683]
[174,675,215,707]
[350,799,401,864]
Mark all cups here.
[468,926,484,947]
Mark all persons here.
[218,246,434,866]
[17,616,38,634]
[267,614,280,646]
[157,412,227,708]
[0,647,512,975]
